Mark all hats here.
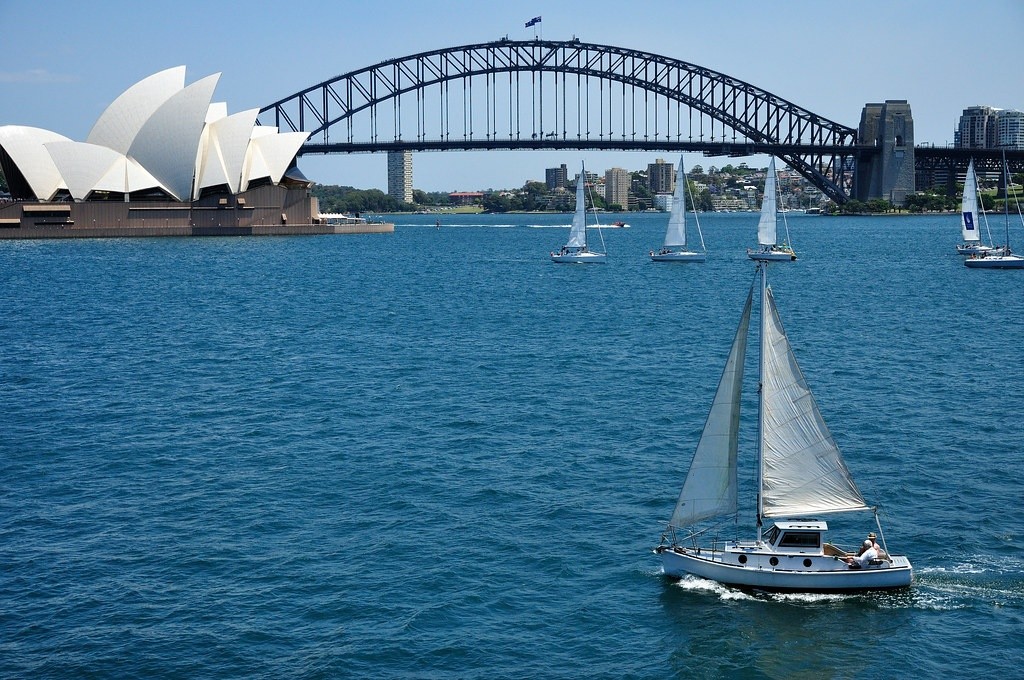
[867,532,877,538]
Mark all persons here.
[660,248,671,255]
[847,533,880,568]
[561,246,570,255]
[765,247,768,251]
[577,249,580,252]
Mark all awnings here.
[238,198,245,204]
[0,218,21,223]
[23,205,70,211]
[220,199,227,204]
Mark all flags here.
[525,16,541,27]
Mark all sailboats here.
[653,258,913,593]
[649,154,707,263]
[550,160,608,264]
[746,153,798,261]
[964,147,1024,268]
[956,156,996,255]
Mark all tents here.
[318,212,347,223]
